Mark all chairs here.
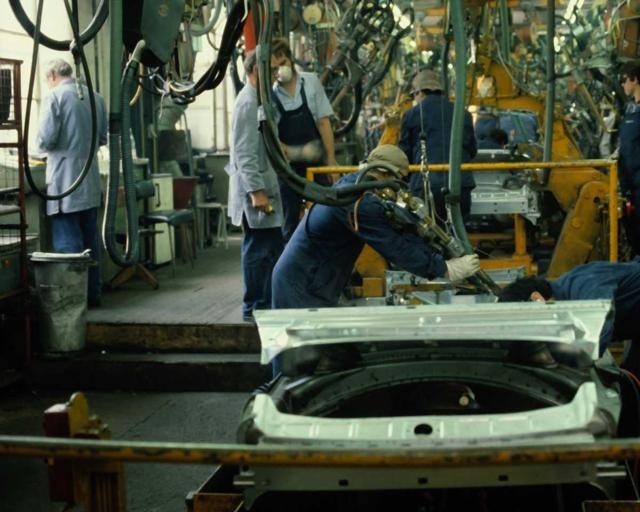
[135,179,198,272]
[195,202,246,253]
[99,186,167,291]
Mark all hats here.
[409,69,443,96]
[366,143,410,181]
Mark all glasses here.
[621,75,631,83]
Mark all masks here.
[272,65,292,84]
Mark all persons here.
[268,34,343,245]
[499,259,640,446]
[397,66,477,241]
[228,49,288,325]
[32,55,110,312]
[612,55,640,260]
[267,142,486,451]
[470,107,508,149]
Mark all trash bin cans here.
[28,249,95,353]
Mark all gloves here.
[443,252,482,284]
